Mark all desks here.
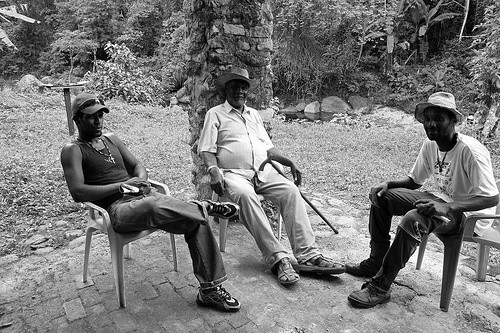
[51,86,85,136]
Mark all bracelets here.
[207,166,217,172]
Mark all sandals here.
[277,258,300,284]
[299,254,346,274]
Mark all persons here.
[197,67,347,284]
[344,92,500,308]
[60,94,242,312]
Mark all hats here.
[72,94,109,119]
[216,67,259,98]
[414,92,465,125]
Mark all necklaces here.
[436,146,449,172]
[77,136,115,164]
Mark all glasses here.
[76,99,104,116]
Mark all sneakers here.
[196,285,241,312]
[200,199,240,219]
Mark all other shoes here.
[344,259,378,278]
[348,282,391,307]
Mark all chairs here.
[212,160,284,253]
[81,178,181,310]
[415,179,500,312]
